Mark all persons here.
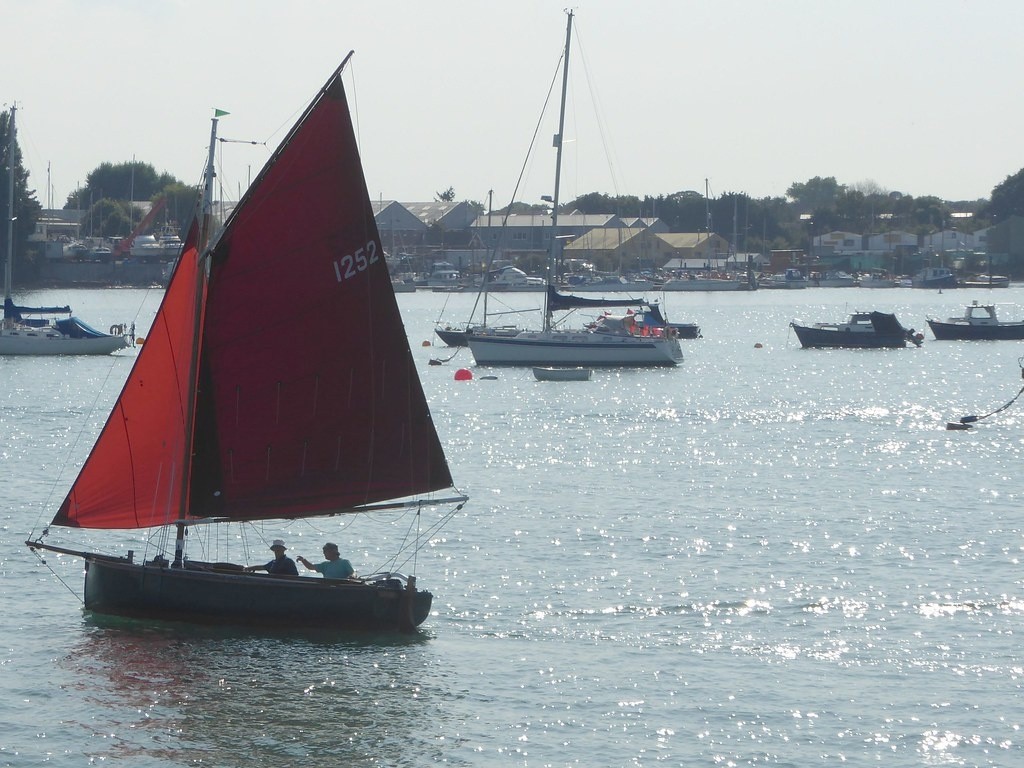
[246,539,299,577]
[297,543,354,579]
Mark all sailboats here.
[0,100,137,356]
[22,49,469,630]
[390,179,1011,293]
[635,303,699,338]
[435,188,543,346]
[463,7,685,366]
[27,154,186,259]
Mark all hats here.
[323,543,340,555]
[270,540,286,552]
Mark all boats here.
[925,299,1024,340]
[789,309,924,347]
[533,367,593,381]
